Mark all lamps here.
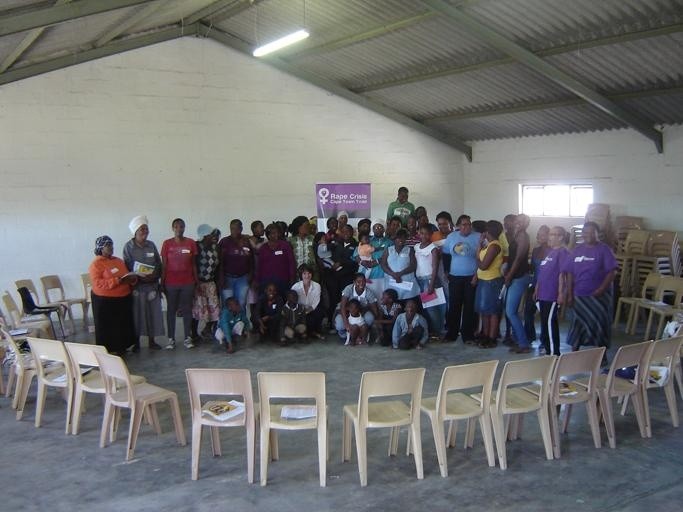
[252,0,310,58]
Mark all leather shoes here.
[466,333,487,345]
[479,337,497,348]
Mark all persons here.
[288,264,320,319]
[345,299,368,346]
[432,211,454,311]
[353,217,394,299]
[337,211,348,233]
[371,287,401,346]
[476,221,505,348]
[274,221,289,240]
[254,224,297,290]
[123,215,162,353]
[308,215,317,237]
[504,215,515,243]
[381,229,421,306]
[406,205,429,245]
[333,273,378,337]
[357,218,371,233]
[216,219,252,311]
[287,216,320,284]
[336,225,357,258]
[566,222,619,367]
[213,296,250,353]
[89,236,136,355]
[325,218,339,239]
[505,215,530,352]
[386,216,402,237]
[471,220,487,232]
[248,221,268,320]
[132,260,154,277]
[192,228,220,344]
[251,282,287,345]
[160,219,201,349]
[317,231,343,271]
[120,273,145,281]
[387,186,414,228]
[533,226,570,355]
[281,291,311,344]
[358,231,385,284]
[391,299,429,350]
[442,214,486,345]
[412,224,443,341]
[523,225,554,342]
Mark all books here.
[10,328,31,335]
[559,381,577,396]
[421,286,446,308]
[650,366,669,385]
[420,289,438,303]
[281,407,316,419]
[53,368,92,382]
[20,314,45,322]
[388,279,413,290]
[204,400,244,422]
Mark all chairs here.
[601,335,683,438]
[561,340,653,449]
[513,346,606,459]
[257,372,329,488]
[92,350,186,461]
[0,309,48,339]
[39,275,88,334]
[0,339,34,413]
[2,290,53,340]
[617,326,683,416]
[516,203,683,340]
[1,328,64,420]
[26,336,99,434]
[17,287,65,340]
[15,279,68,337]
[341,368,426,487]
[406,359,499,478]
[185,368,272,483]
[80,272,91,322]
[464,354,557,470]
[64,342,149,442]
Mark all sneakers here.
[183,336,194,349]
[166,338,176,349]
